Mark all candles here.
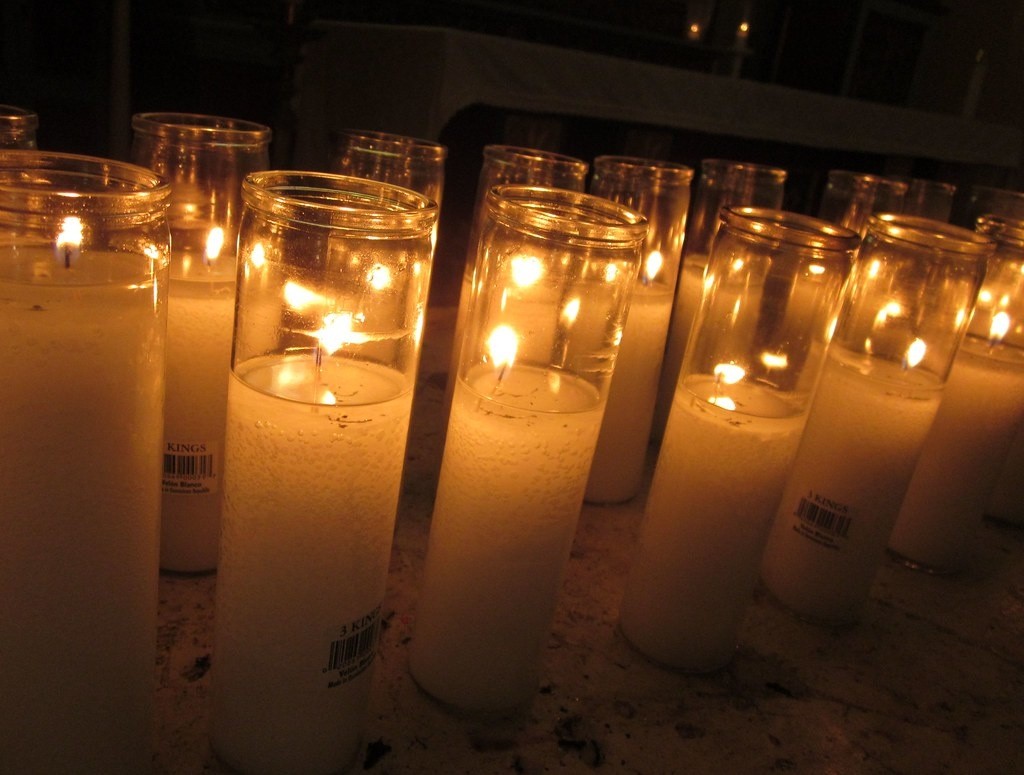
[447,253,559,428]
[887,310,1024,576]
[164,225,282,572]
[410,321,605,721]
[654,250,774,446]
[0,217,168,774]
[760,339,946,621]
[617,362,806,674]
[210,307,414,775]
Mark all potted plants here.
[559,250,675,503]
